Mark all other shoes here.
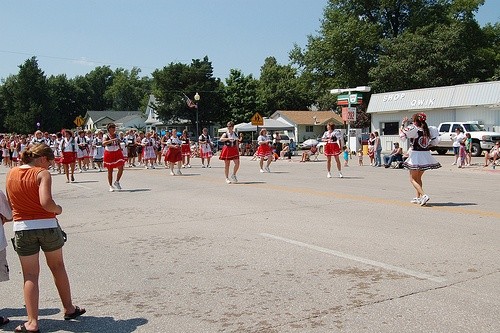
[114,181,121,189]
[50,161,191,176]
[109,186,115,191]
[71,175,75,181]
[265,166,270,173]
[338,172,343,178]
[231,175,238,183]
[66,178,69,183]
[225,178,232,184]
[420,195,430,206]
[0,316,10,326]
[202,164,211,168]
[328,173,332,178]
[411,197,421,204]
[260,169,265,172]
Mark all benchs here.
[285,145,324,160]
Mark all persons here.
[452,127,473,168]
[484,141,500,167]
[5,143,86,333]
[0,188,14,328]
[399,113,443,206]
[0,121,403,192]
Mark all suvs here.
[429,121,500,157]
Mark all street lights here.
[193,92,201,138]
[330,86,371,153]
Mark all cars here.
[359,132,372,146]
[298,139,319,149]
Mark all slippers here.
[63,305,86,320]
[14,323,40,333]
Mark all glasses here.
[47,159,52,167]
